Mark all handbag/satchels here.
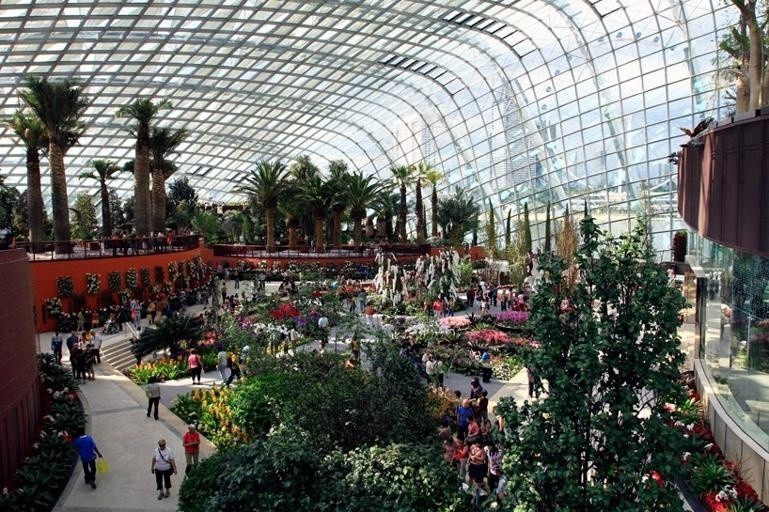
[146,389,152,398]
[170,463,174,472]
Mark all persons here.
[480,347,493,383]
[527,363,540,399]
[145,376,160,420]
[129,264,267,332]
[278,270,455,355]
[74,428,103,489]
[442,376,515,497]
[189,345,248,387]
[151,439,177,500]
[51,303,124,383]
[183,424,200,463]
[422,349,444,388]
[467,279,527,312]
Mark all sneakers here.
[158,490,170,500]
[84,480,98,489]
